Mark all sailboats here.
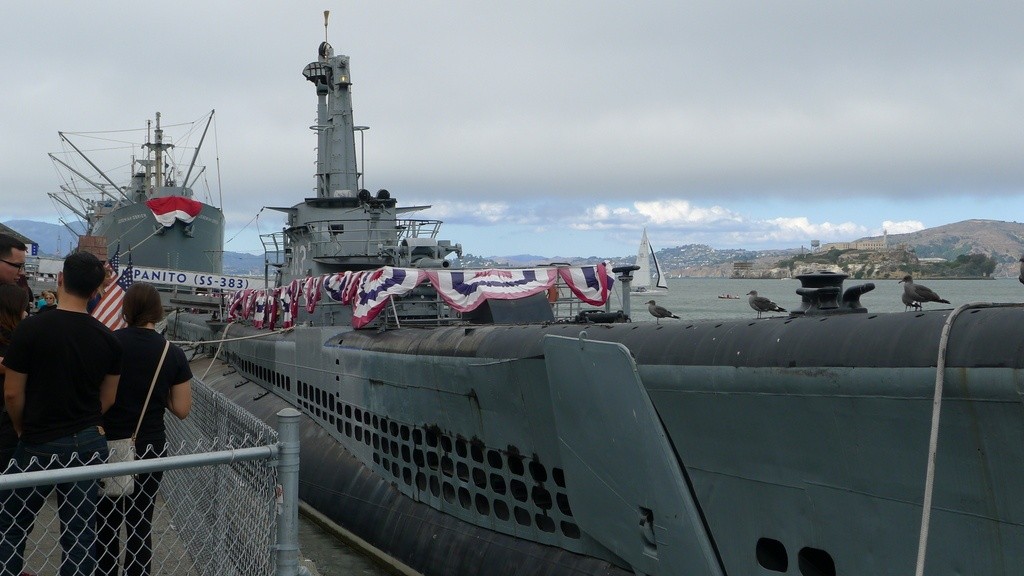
[629,225,669,297]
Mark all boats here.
[781,277,791,280]
[718,294,740,299]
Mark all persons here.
[0,233,57,576]
[2,251,122,576]
[95,280,196,576]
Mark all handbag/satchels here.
[98,438,136,497]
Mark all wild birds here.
[898,274,951,312]
[747,290,787,319]
[644,299,680,325]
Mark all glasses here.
[1,259,25,271]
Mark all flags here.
[90,253,139,334]
[89,243,122,315]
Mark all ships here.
[169,38,1024,576]
[48,110,226,308]
